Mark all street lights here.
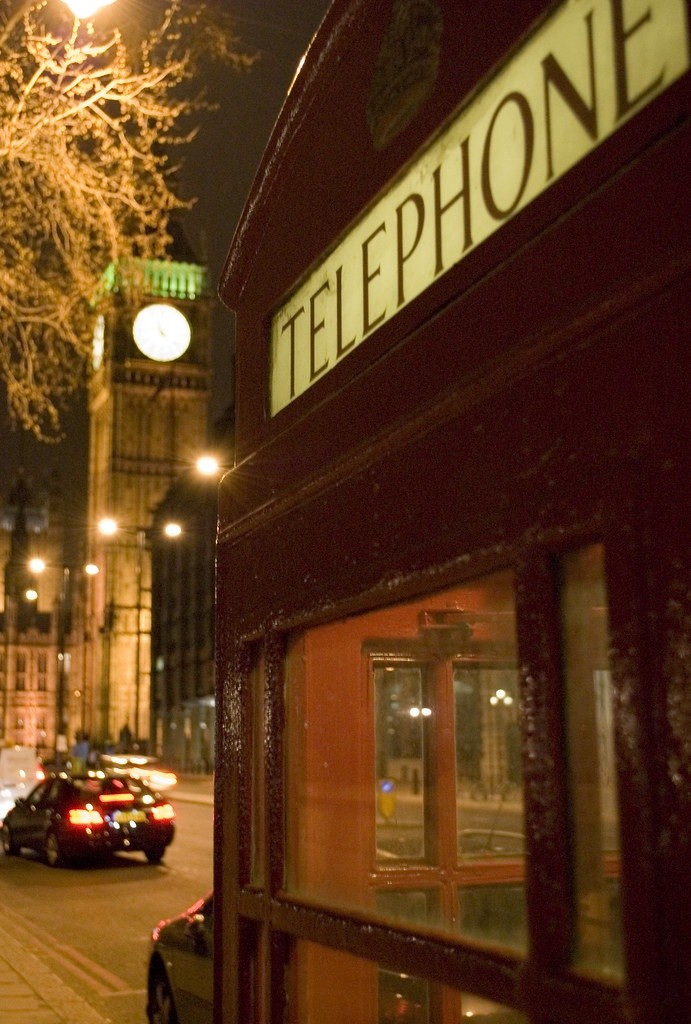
[98,517,181,741]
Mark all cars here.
[0,770,176,871]
[148,834,454,1024]
